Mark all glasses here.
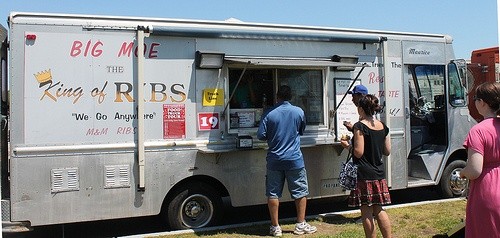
[473,95,481,101]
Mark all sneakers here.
[269,225,282,237]
[293,220,317,235]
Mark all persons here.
[257,85,317,237]
[459,81,500,238]
[338,84,392,238]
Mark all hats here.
[348,84,368,94]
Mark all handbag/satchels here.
[338,147,357,190]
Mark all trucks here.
[1,10,483,234]
